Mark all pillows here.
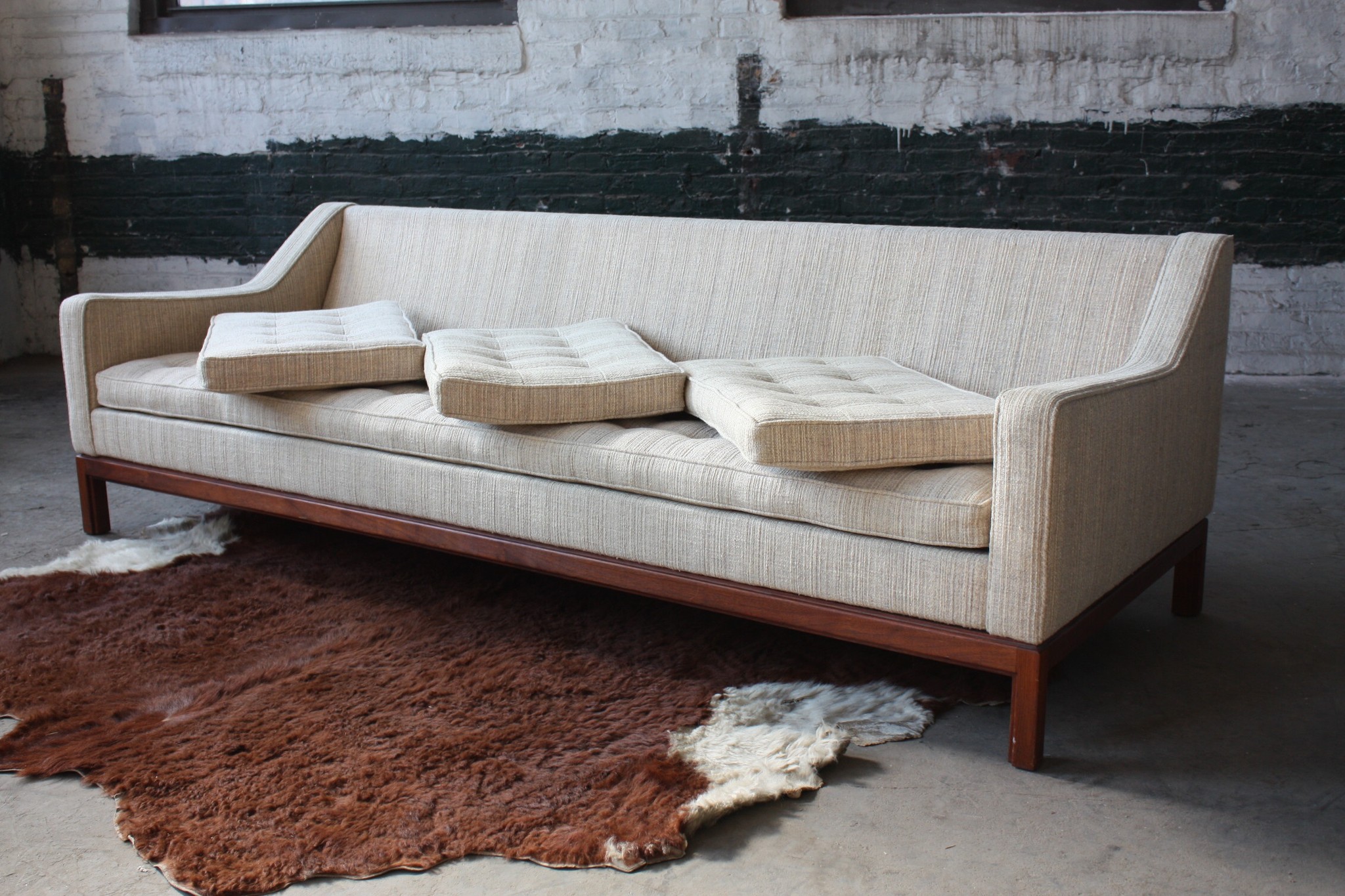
[424,318,686,428]
[199,301,425,407]
[670,359,999,473]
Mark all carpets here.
[0,501,1015,896]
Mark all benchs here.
[63,198,1239,770]
